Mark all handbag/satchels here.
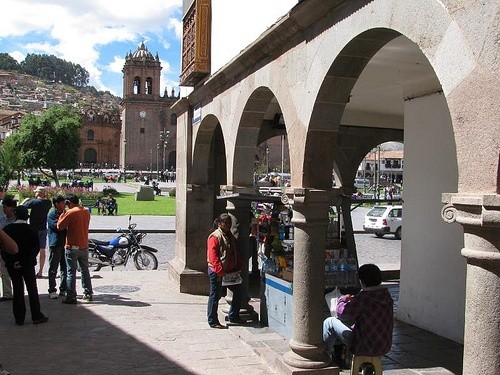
[222,271,243,286]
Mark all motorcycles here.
[76,213,158,273]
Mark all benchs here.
[72,184,93,191]
[81,199,118,214]
[30,181,51,186]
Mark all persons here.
[47,194,67,299]
[1,204,50,325]
[383,185,395,199]
[356,192,364,206]
[71,178,93,191]
[28,177,49,185]
[0,227,19,253]
[94,195,106,216]
[207,213,247,328]
[57,193,93,304]
[106,193,116,215]
[323,263,394,368]
[78,160,175,195]
[0,186,52,301]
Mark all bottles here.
[323,253,358,289]
[250,215,294,242]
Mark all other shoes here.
[16,319,24,326]
[33,317,49,324]
[230,318,246,324]
[0,297,13,302]
[62,298,76,303]
[83,294,93,301]
[37,273,42,278]
[211,324,226,329]
[59,291,65,296]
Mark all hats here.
[33,186,46,193]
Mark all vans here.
[354,178,371,189]
[259,172,291,187]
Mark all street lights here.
[10,115,22,185]
[266,148,269,174]
[123,139,127,183]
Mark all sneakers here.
[49,292,59,299]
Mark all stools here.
[350,355,383,375]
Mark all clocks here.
[139,111,146,118]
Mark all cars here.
[363,204,402,240]
[380,174,387,179]
